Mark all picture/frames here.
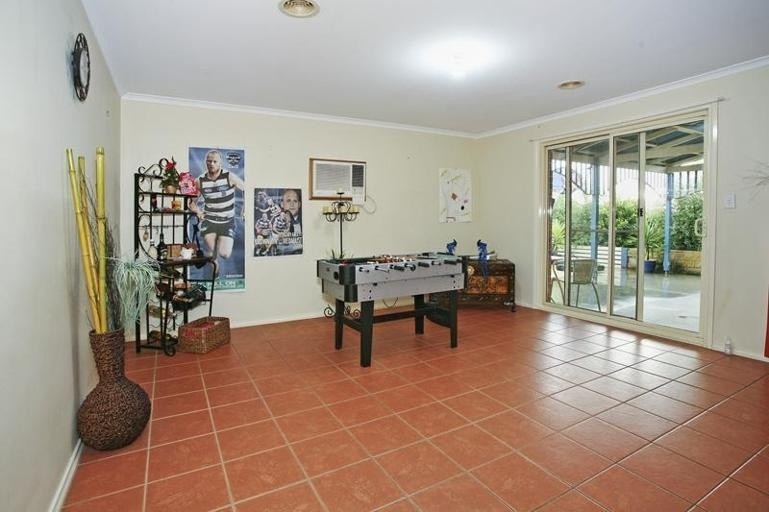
[308,157,367,201]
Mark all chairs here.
[562,260,601,311]
[551,260,565,304]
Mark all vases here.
[167,185,177,193]
[78,325,154,448]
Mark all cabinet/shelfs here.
[429,252,516,314]
[133,159,217,357]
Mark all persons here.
[255,190,267,208]
[280,189,301,227]
[187,150,244,277]
[257,197,282,219]
[273,212,290,243]
[255,214,273,255]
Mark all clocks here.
[70,32,91,102]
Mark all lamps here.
[322,187,360,259]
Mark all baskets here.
[178,316,230,354]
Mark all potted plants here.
[624,215,663,272]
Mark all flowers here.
[160,158,181,189]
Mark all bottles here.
[148,239,157,260]
[157,234,167,262]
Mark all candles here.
[340,206,347,212]
[349,207,354,212]
[353,205,360,212]
[328,206,333,212]
[338,187,343,192]
[323,206,328,213]
